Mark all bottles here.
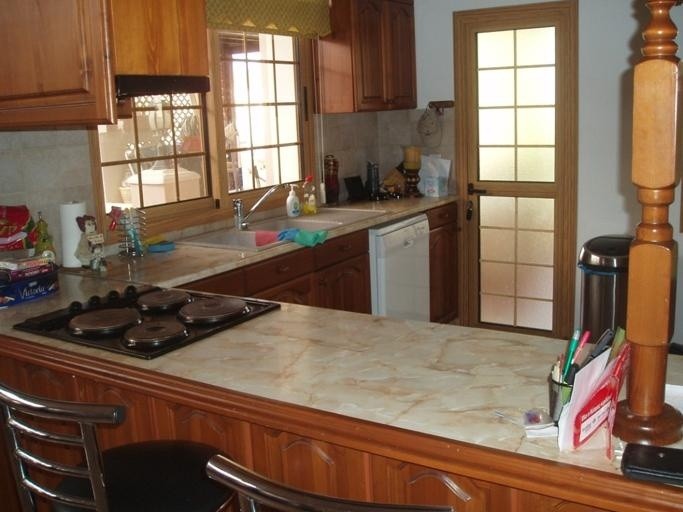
[320,153,341,206]
[367,163,380,200]
[34,211,53,260]
[302,176,319,215]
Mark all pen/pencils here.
[552,327,625,424]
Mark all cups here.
[548,376,573,425]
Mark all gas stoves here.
[12,284,282,363]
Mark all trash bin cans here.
[576,235,634,347]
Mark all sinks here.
[248,220,343,230]
[173,231,291,251]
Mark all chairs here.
[0,382,237,511]
[204,455,455,512]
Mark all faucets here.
[232,184,288,231]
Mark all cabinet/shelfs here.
[0,1,212,131]
[317,0,418,116]
[176,228,373,346]
[426,200,457,324]
